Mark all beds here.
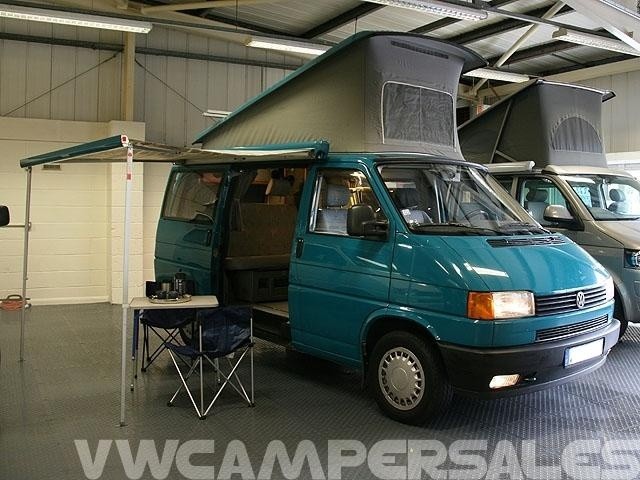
[128,294,221,393]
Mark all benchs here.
[221,175,298,270]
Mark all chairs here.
[393,187,433,226]
[607,189,628,213]
[164,303,255,421]
[346,204,377,239]
[315,184,351,235]
[523,189,554,225]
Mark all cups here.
[156,290,178,299]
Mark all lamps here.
[463,68,530,83]
[0,3,152,34]
[552,26,640,56]
[364,0,488,21]
[243,35,333,56]
[203,109,232,119]
